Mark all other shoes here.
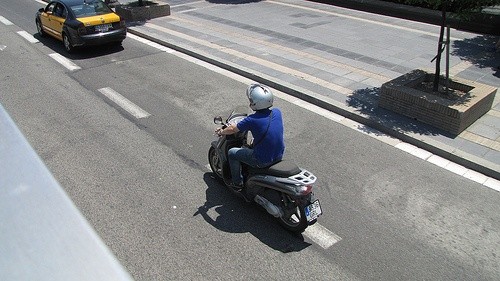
[230,180,245,190]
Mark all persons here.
[214,83,285,188]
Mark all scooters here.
[208,106,323,234]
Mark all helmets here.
[246,84,273,112]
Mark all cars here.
[36,0,127,53]
